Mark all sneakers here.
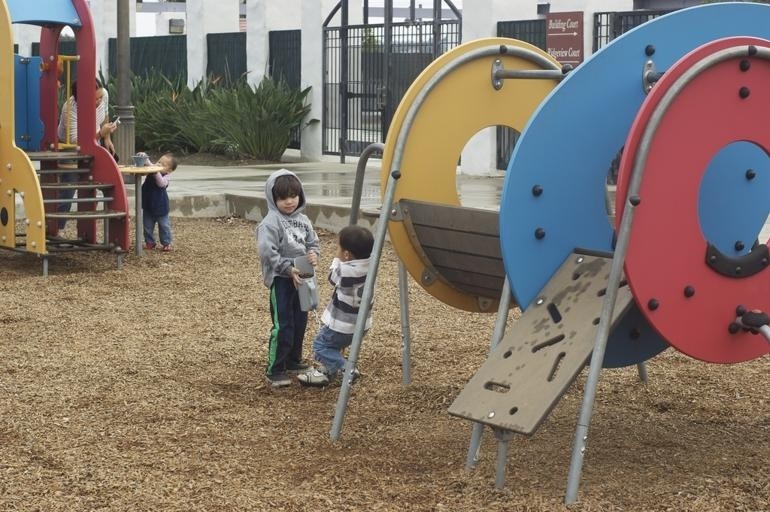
[296,371,330,387]
[162,244,172,251]
[264,373,292,387]
[334,365,360,387]
[57,227,72,241]
[285,360,310,374]
[143,242,157,250]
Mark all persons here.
[136,152,178,251]
[255,169,320,385]
[57,77,116,236]
[297,225,375,386]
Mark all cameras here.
[113,115,121,125]
